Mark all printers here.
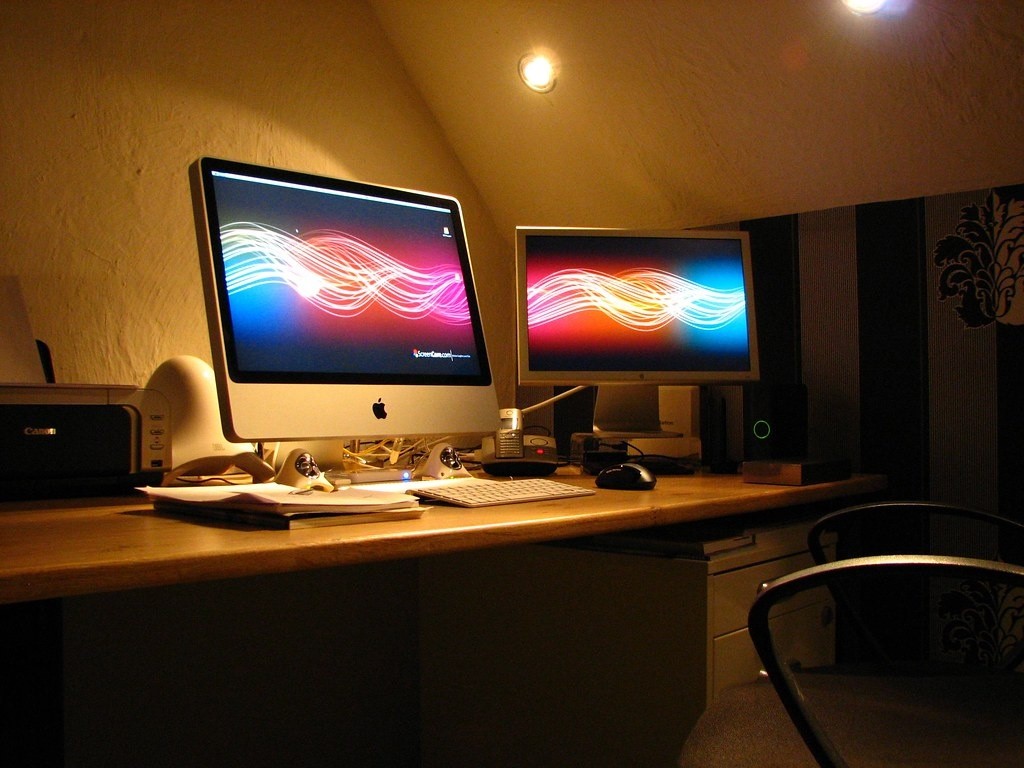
[0,339,172,508]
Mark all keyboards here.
[405,478,596,508]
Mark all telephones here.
[480,405,560,476]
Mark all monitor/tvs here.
[187,155,503,490]
[513,224,760,439]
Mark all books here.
[154,503,424,530]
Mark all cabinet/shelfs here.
[419,515,838,768]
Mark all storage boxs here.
[742,459,853,486]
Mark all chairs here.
[676,501,1024,768]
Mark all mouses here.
[595,463,656,490]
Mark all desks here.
[0,471,887,768]
